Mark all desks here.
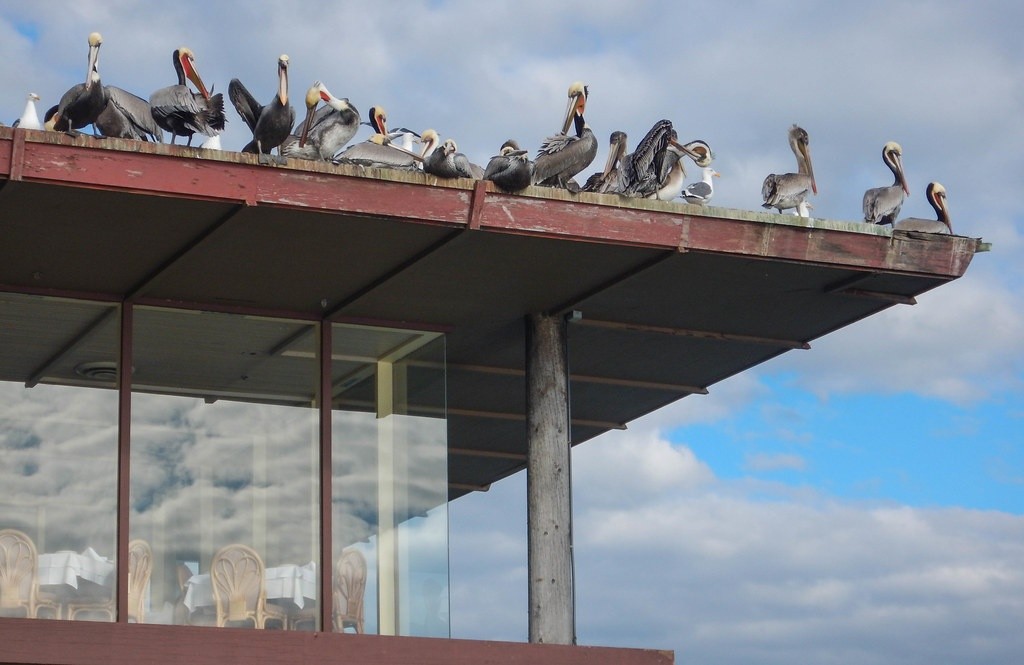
[183,564,316,613]
[38,552,114,620]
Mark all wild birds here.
[280,79,361,163]
[44,31,165,144]
[531,81,598,193]
[582,117,720,206]
[894,181,954,234]
[227,54,297,166]
[863,140,910,229]
[148,47,228,148]
[760,121,817,216]
[481,138,537,192]
[11,91,43,130]
[333,104,483,180]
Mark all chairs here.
[210,543,288,630]
[176,565,208,626]
[66,539,154,624]
[0,528,64,618]
[290,548,367,634]
